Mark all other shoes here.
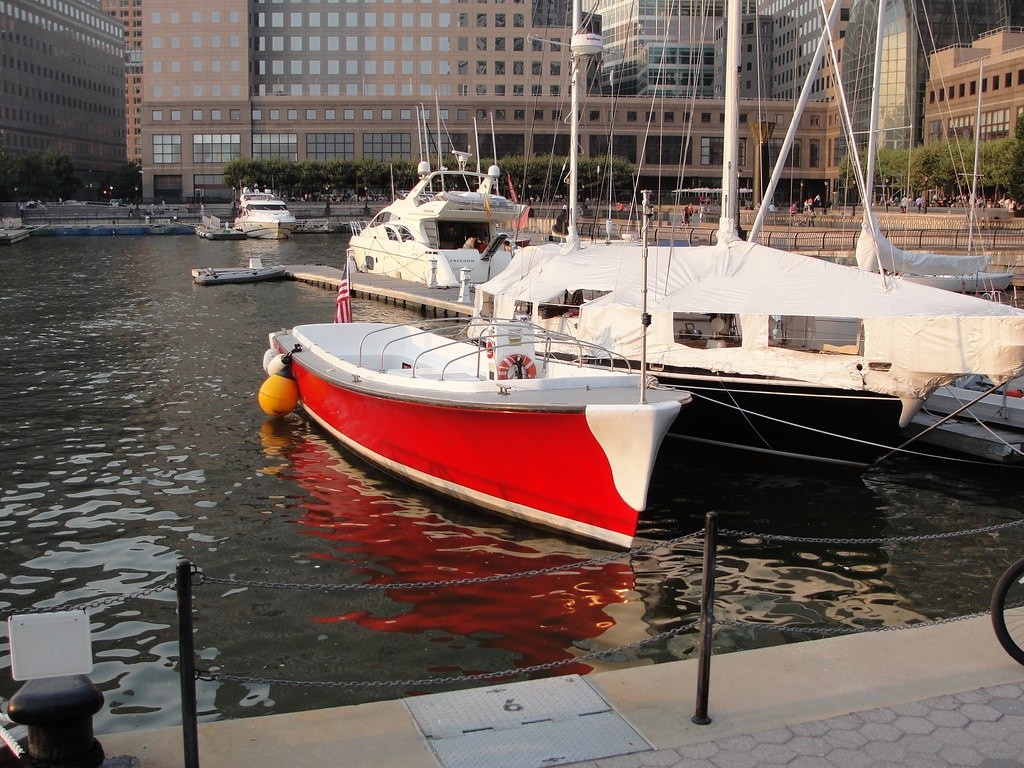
[902,211,906,213]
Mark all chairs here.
[819,324,863,355]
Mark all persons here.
[18,204,26,219]
[128,206,135,216]
[462,232,480,249]
[739,193,822,226]
[680,196,720,229]
[506,191,636,218]
[880,194,1015,213]
[279,191,399,202]
[503,240,512,251]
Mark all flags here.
[333,260,352,323]
[483,192,492,218]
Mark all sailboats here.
[346,0,1024,427]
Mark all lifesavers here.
[497,354,538,380]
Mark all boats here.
[254,187,693,554]
[233,186,297,240]
